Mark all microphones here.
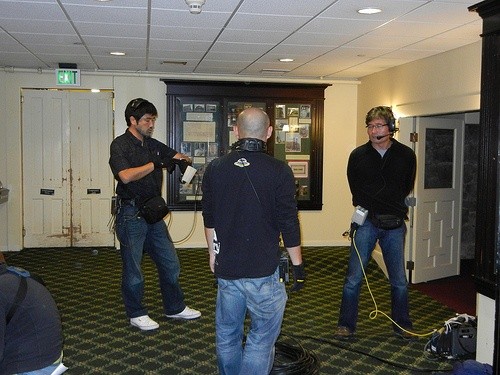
[377,133,390,140]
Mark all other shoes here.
[335,326,350,339]
[402,329,414,339]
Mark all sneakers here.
[167,305,202,319]
[130,315,159,330]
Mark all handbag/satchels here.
[140,196,169,224]
[373,213,403,230]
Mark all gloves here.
[154,158,182,174]
[291,263,306,292]
[179,159,197,183]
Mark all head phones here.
[386,107,395,131]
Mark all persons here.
[334,106,417,339]
[109,98,202,331]
[0,250,68,375]
[200,107,307,375]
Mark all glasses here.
[132,98,144,118]
[366,123,389,130]
